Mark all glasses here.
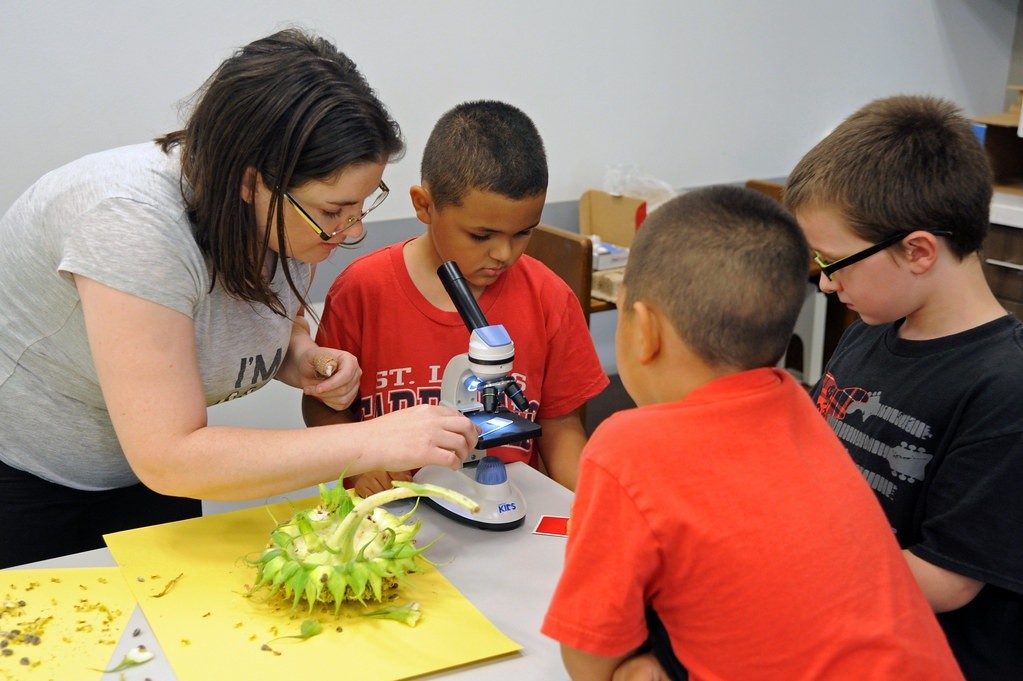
[815,230,952,281]
[273,180,390,241]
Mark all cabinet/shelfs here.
[978,223,1023,323]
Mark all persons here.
[302,99,610,495]
[0,30,482,569]
[782,99,1023,681]
[539,190,969,680]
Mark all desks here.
[525,181,827,429]
[1,460,575,681]
